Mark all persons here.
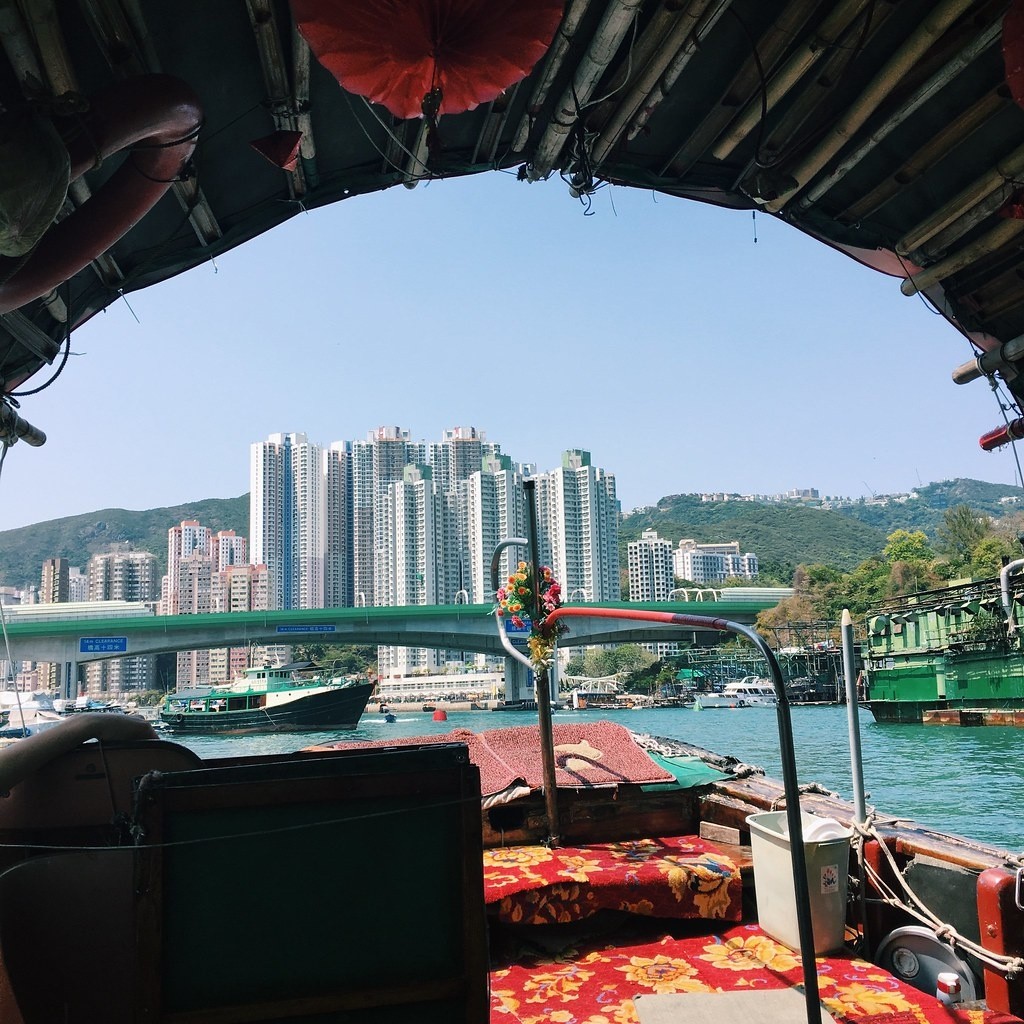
[0,712,160,794]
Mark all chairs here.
[0,738,206,1024]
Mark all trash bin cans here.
[745,808,856,957]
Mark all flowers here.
[482,561,571,673]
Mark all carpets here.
[333,721,672,798]
[482,834,743,925]
[485,924,1024,1024]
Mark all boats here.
[855,556,1023,729]
[381,703,389,714]
[423,704,436,713]
[0,696,175,740]
[566,676,780,710]
[384,714,397,723]
[158,678,379,735]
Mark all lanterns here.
[285,0,567,119]
